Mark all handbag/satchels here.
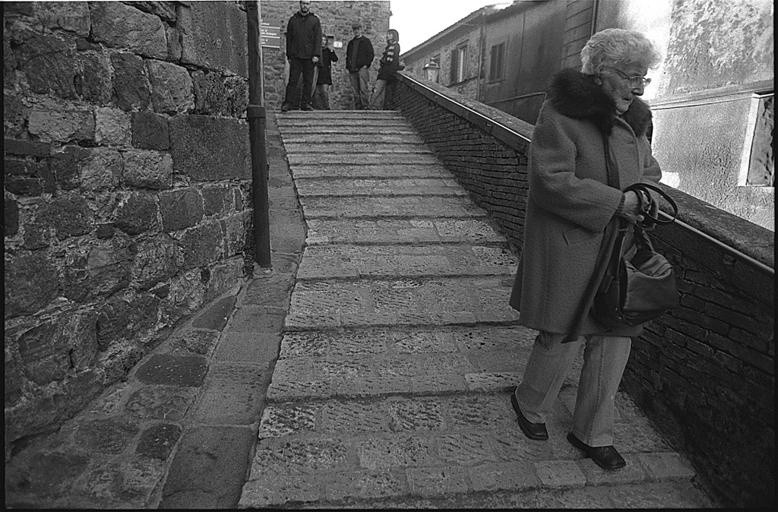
[590,224,676,328]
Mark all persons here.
[345,22,375,110]
[281,1,322,111]
[507,26,663,472]
[369,28,401,110]
[282,27,339,111]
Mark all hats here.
[351,24,362,29]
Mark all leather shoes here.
[281,103,330,111]
[567,432,625,469]
[511,393,549,441]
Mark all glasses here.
[614,68,651,89]
